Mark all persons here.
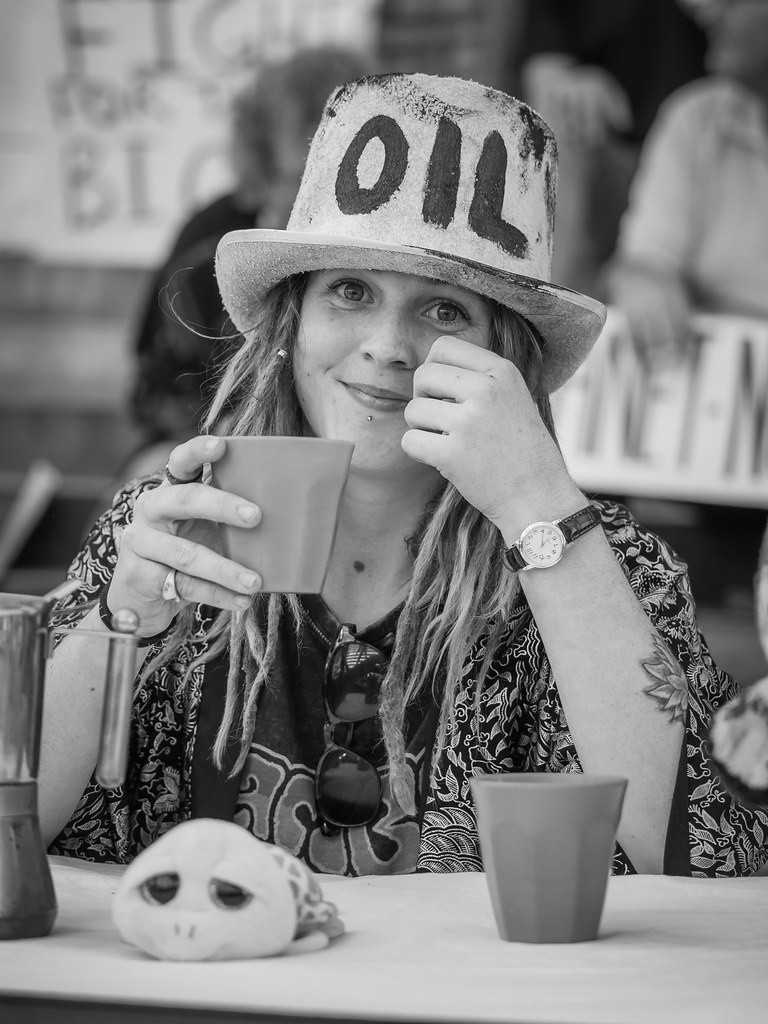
[126,49,372,487]
[531,1,768,607]
[38,73,768,879]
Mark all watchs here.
[506,504,602,571]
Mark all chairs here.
[562,313,768,508]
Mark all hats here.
[214,72,607,395]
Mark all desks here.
[0,853,768,1024]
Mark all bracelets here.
[99,577,179,648]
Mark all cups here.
[0,581,140,940]
[209,434,355,594]
[468,773,628,945]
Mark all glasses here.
[314,622,390,828]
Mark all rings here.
[165,460,204,485]
[162,569,183,602]
